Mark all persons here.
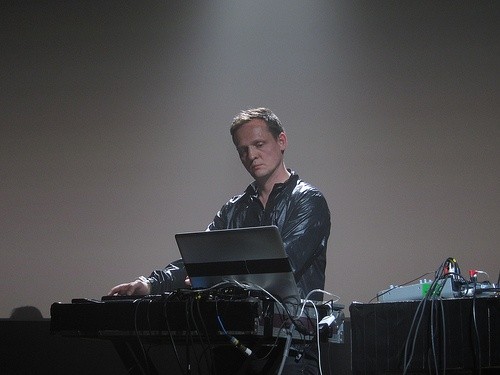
[105,107,333,302]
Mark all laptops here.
[175,225,331,306]
